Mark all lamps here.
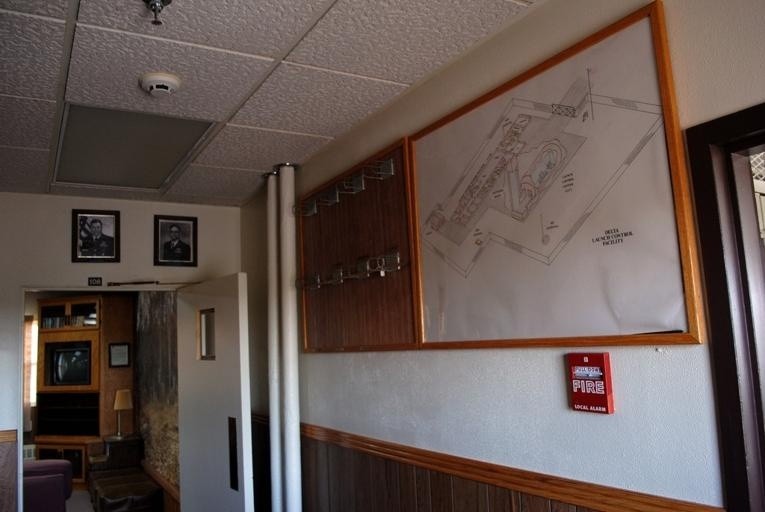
[111,389,134,439]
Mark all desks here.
[103,436,143,468]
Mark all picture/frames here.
[72,209,121,263]
[407,0,706,351]
[154,215,198,266]
[108,343,130,368]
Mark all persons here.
[164,225,191,261]
[82,219,113,256]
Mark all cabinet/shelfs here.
[33,293,137,485]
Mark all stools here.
[86,466,164,512]
[88,455,108,471]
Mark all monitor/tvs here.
[54,348,89,385]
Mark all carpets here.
[65,489,94,512]
